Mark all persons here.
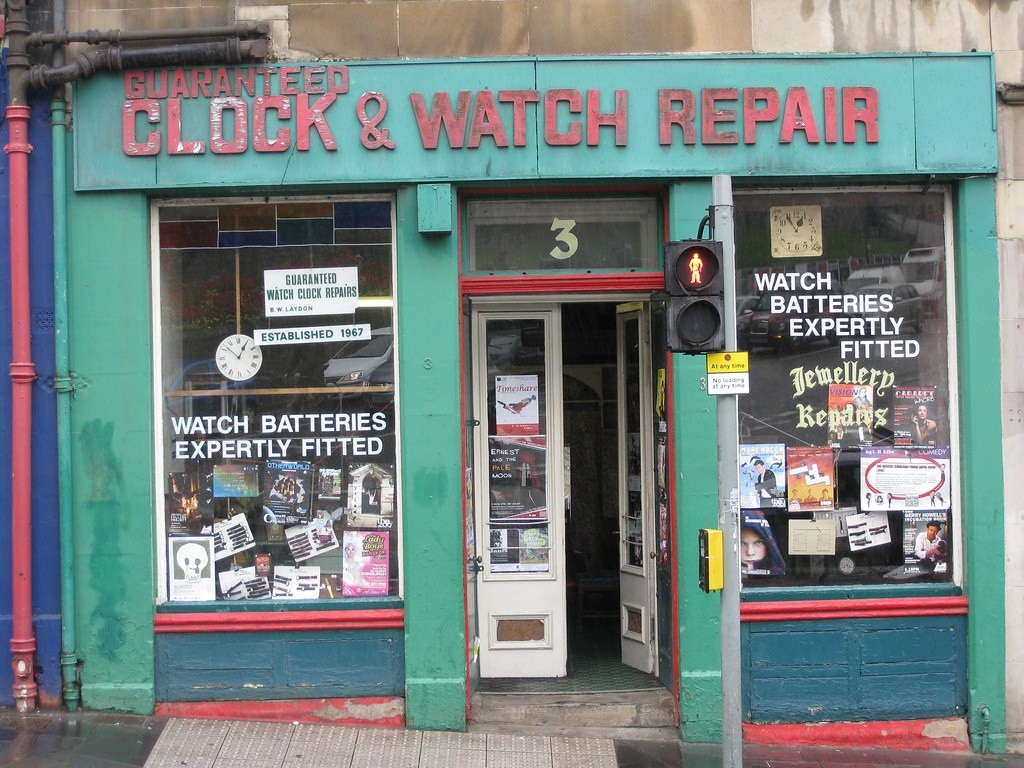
[915,520,948,573]
[840,387,872,447]
[789,489,803,502]
[911,404,937,445]
[748,460,777,507]
[804,489,817,502]
[819,489,833,501]
[741,511,786,576]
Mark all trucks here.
[901,244,946,318]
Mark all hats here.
[927,521,941,530]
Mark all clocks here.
[215,335,264,381]
[768,205,824,258]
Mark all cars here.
[323,327,503,405]
[854,282,926,334]
[845,267,902,295]
[818,279,844,300]
[162,357,309,423]
[736,296,760,352]
[742,280,840,357]
